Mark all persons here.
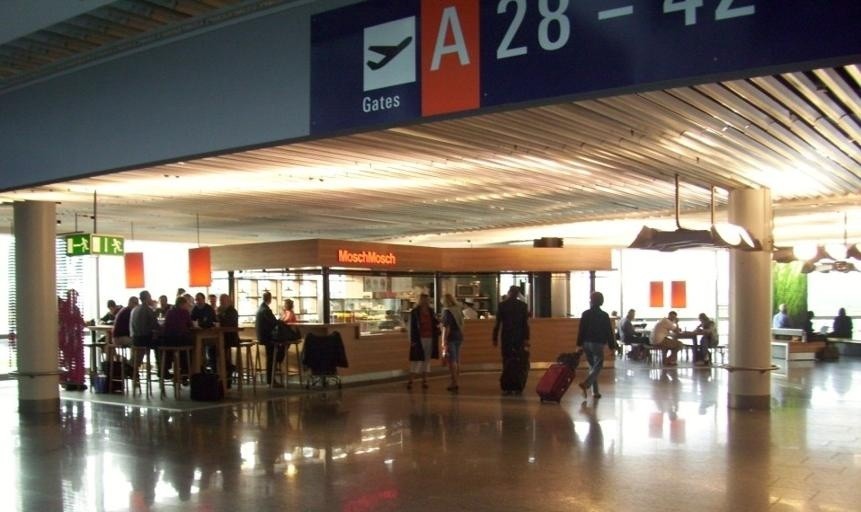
[833,306,855,341]
[609,312,621,339]
[618,308,649,360]
[649,310,685,365]
[56,286,297,389]
[804,310,828,361]
[770,303,802,341]
[492,286,532,396]
[406,292,441,389]
[575,290,620,399]
[769,350,856,409]
[692,312,720,367]
[438,294,466,391]
[620,356,721,422]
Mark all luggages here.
[535,364,576,403]
[501,343,529,393]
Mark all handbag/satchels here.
[557,352,580,370]
[101,359,137,378]
[273,319,302,341]
[190,367,224,401]
[93,374,125,395]
[820,342,840,361]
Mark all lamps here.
[123,220,144,288]
[188,212,212,286]
[626,173,765,253]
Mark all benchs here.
[771,327,861,362]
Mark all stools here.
[83,321,342,403]
[617,329,726,365]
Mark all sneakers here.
[421,383,428,388]
[156,370,190,385]
[446,384,459,391]
[591,392,601,397]
[578,383,588,398]
[407,382,413,391]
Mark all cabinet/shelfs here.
[235,277,318,322]
